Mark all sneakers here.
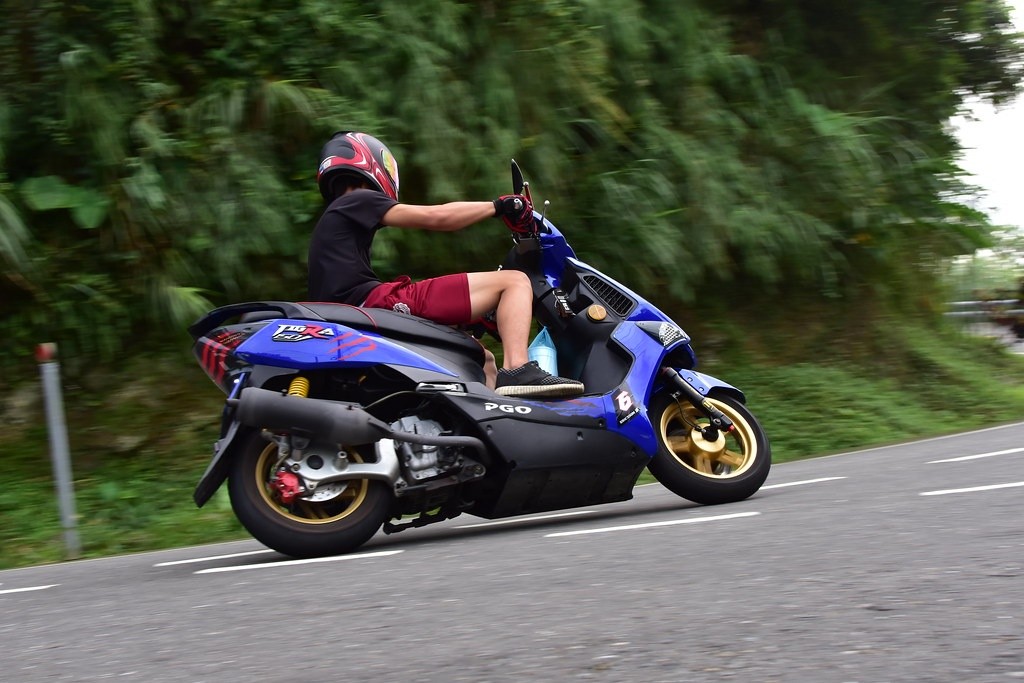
[494,360,584,396]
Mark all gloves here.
[492,194,538,233]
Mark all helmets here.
[317,131,399,202]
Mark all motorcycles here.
[183,159,772,559]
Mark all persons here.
[308,132,585,397]
